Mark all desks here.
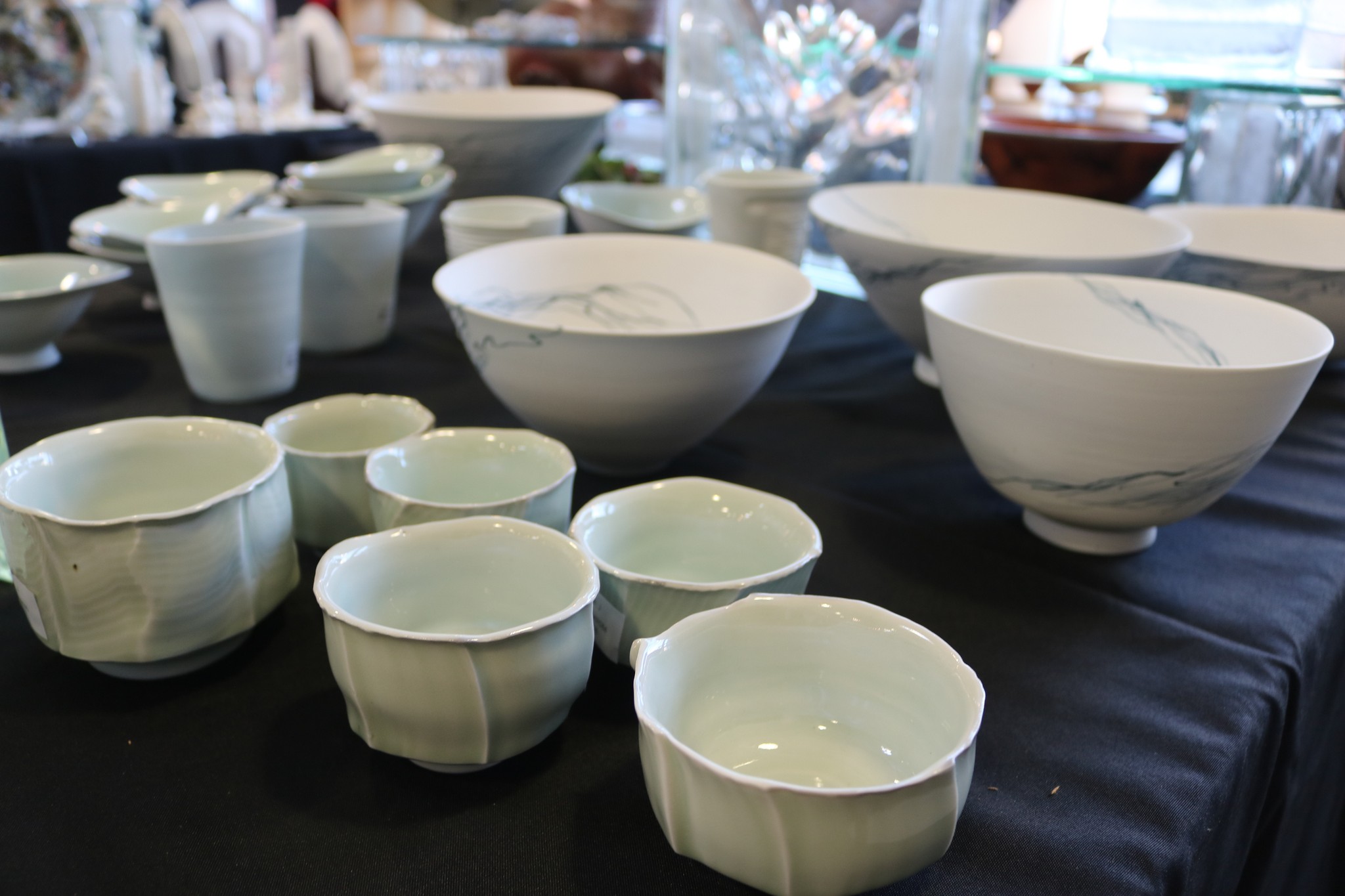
[0,122,1345,896]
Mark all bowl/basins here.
[283,142,455,249]
[975,113,1185,209]
[922,275,1333,553]
[0,417,300,680]
[561,179,710,237]
[430,235,818,459]
[366,88,620,198]
[807,179,1186,387]
[71,171,286,308]
[567,475,822,666]
[262,391,434,547]
[630,589,986,896]
[1152,203,1345,374]
[312,514,600,773]
[362,425,576,537]
[0,254,128,375]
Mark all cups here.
[440,196,566,261]
[713,168,813,269]
[141,221,306,400]
[251,203,408,347]
